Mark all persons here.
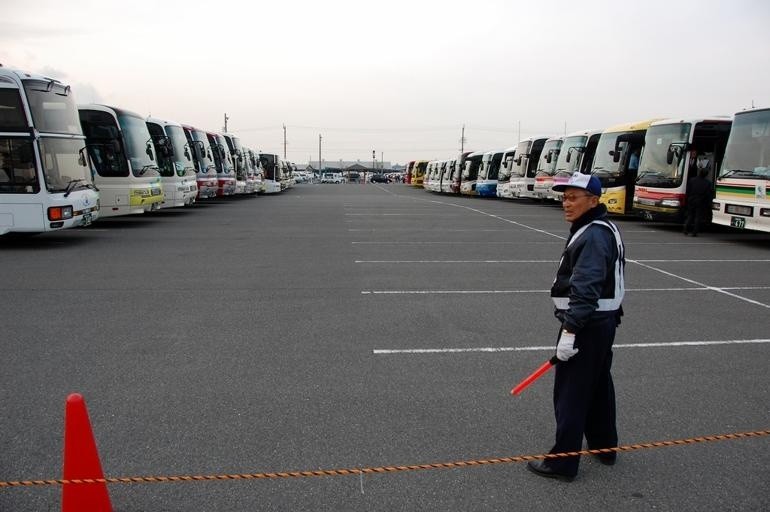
[392,173,401,184]
[358,175,361,184]
[528,172,626,483]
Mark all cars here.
[298,170,404,185]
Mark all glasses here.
[562,193,593,202]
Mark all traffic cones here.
[58,393,116,511]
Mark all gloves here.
[556,328,580,363]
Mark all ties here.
[691,160,693,169]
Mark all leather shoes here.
[526,459,579,483]
[585,442,616,467]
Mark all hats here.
[551,171,603,199]
